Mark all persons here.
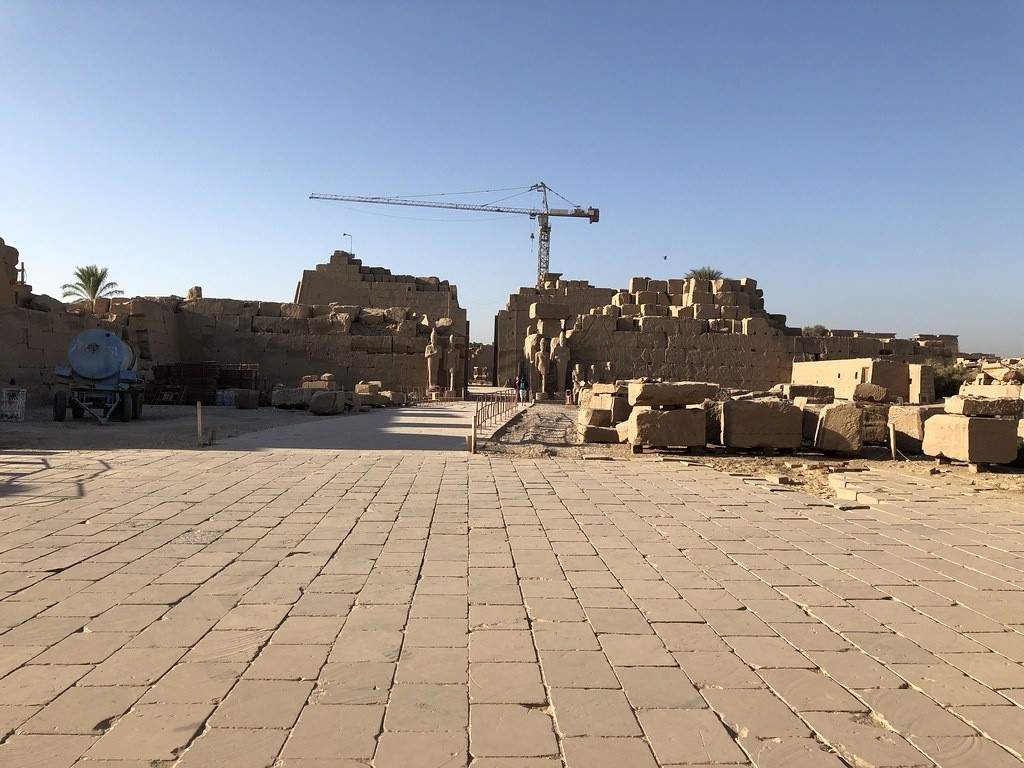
[554,332,570,393]
[443,334,460,392]
[519,375,528,406]
[425,330,442,392]
[534,338,549,394]
[572,362,615,390]
[515,376,520,399]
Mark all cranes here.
[309,181,600,289]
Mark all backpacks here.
[520,379,526,389]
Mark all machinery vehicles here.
[52,329,145,426]
